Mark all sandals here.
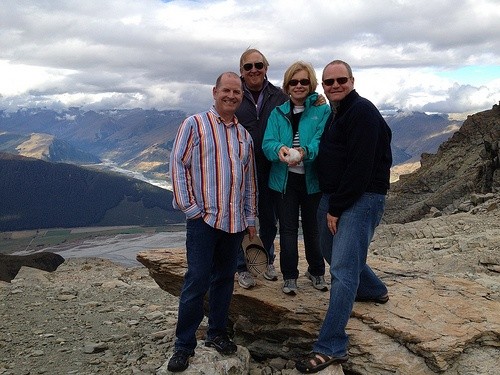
[295,353,349,373]
[354,293,388,303]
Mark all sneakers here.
[237,272,255,288]
[283,277,298,296]
[167,349,194,371]
[306,269,328,290]
[264,265,278,280]
[205,331,238,353]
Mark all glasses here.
[243,62,265,71]
[323,77,351,86]
[288,79,310,86]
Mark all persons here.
[262,61,331,295]
[235,48,326,289]
[167,72,259,372]
[295,60,392,374]
